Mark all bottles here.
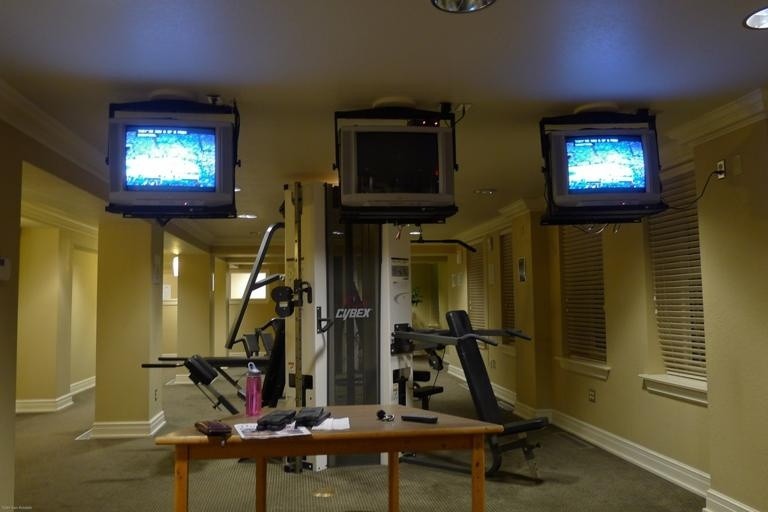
[244,361,262,417]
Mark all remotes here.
[402,416,438,423]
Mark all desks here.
[153,400,507,510]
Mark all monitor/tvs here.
[340,126,454,207]
[548,129,661,208]
[109,117,234,206]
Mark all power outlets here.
[716,160,725,179]
